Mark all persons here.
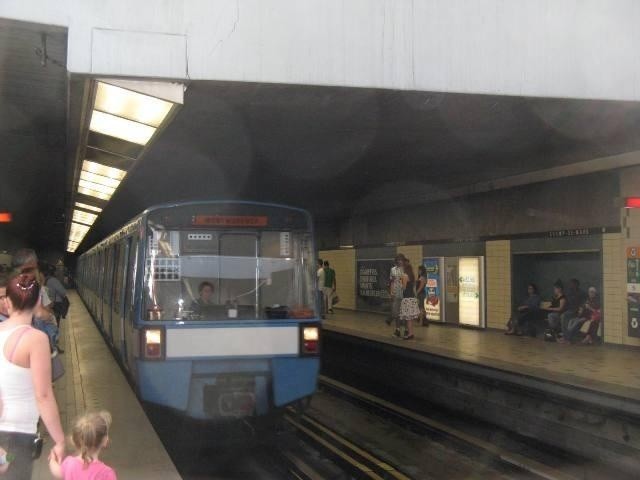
[317,257,326,320]
[384,251,430,339]
[323,260,336,315]
[44,409,116,480]
[191,280,214,305]
[503,276,602,344]
[0,245,68,480]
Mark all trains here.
[68,201,321,418]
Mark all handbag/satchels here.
[52,350,65,382]
[544,328,556,341]
[332,296,338,305]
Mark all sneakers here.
[392,330,413,339]
[328,309,333,314]
[505,333,524,335]
[560,337,592,345]
